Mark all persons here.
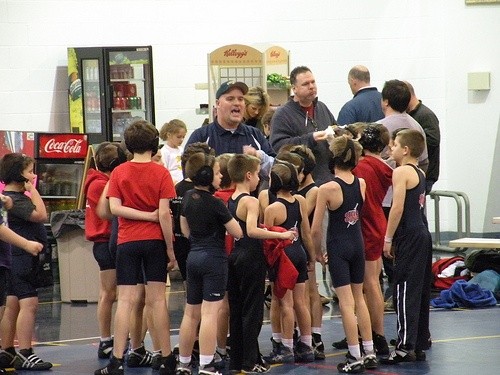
[0,152,54,370]
[174,153,243,375]
[84,64,439,375]
[381,130,433,363]
[312,135,380,375]
[0,190,45,321]
[226,154,298,374]
[94,120,177,375]
[349,124,394,356]
[263,164,312,361]
[168,143,217,362]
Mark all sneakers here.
[198,360,224,375]
[159,358,178,375]
[241,363,272,375]
[380,345,416,363]
[263,346,296,363]
[313,341,325,360]
[374,337,390,355]
[94,359,124,375]
[176,360,193,375]
[415,346,426,362]
[361,345,377,369]
[151,354,162,369]
[214,351,229,373]
[0,345,18,368]
[390,338,398,345]
[295,341,315,363]
[337,352,365,373]
[15,348,52,370]
[98,339,113,358]
[332,338,348,349]
[417,337,432,350]
[127,346,152,367]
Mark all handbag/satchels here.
[465,249,500,273]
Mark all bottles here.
[114,117,144,140]
[43,199,77,222]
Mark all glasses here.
[311,119,320,132]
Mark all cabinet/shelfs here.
[264,45,291,111]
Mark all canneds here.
[39,181,72,196]
[84,65,141,110]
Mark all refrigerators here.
[67,47,154,147]
[0,129,89,291]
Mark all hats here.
[361,123,390,152]
[216,80,248,99]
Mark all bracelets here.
[384,236,393,243]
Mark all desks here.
[449,237,500,249]
